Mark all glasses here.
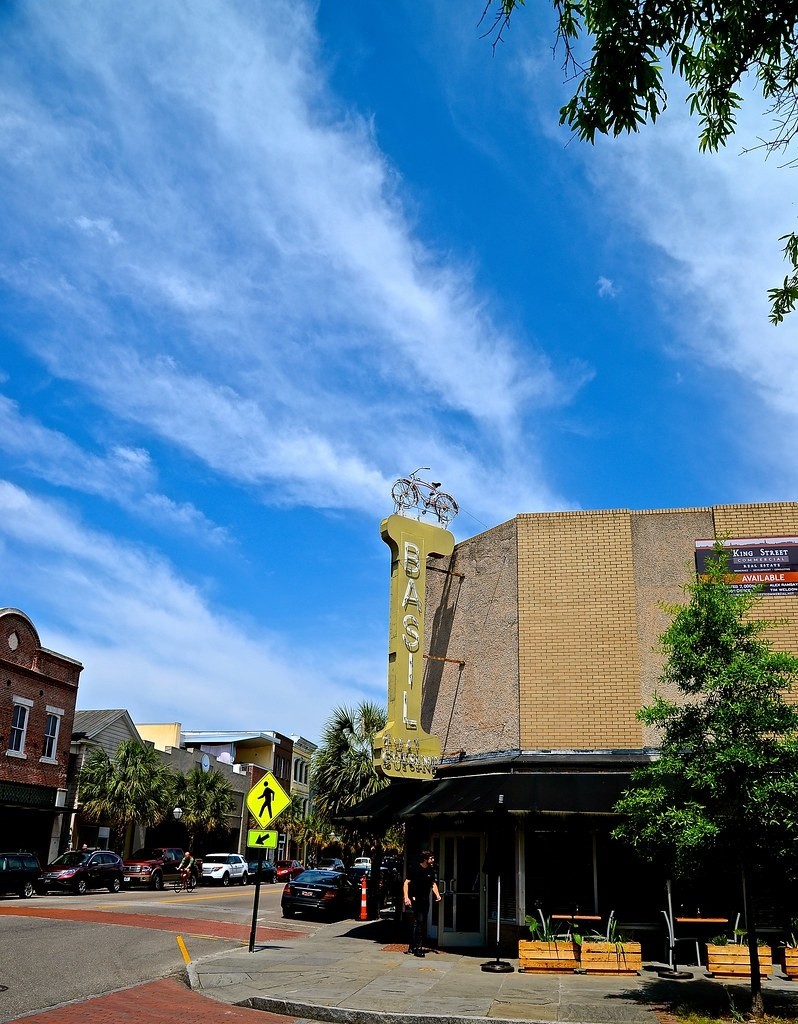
[428,857,434,860]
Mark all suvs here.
[315,858,345,874]
[38,849,125,895]
[0,852,43,899]
[121,847,187,891]
[200,853,249,887]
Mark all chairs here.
[661,911,701,967]
[582,911,615,942]
[537,909,572,942]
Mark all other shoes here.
[414,950,425,957]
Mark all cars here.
[348,857,399,908]
[271,860,305,882]
[247,860,277,885]
[281,868,360,922]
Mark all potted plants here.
[517,915,579,974]
[580,917,642,976]
[777,933,798,982]
[706,930,773,981]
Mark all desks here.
[553,916,601,937]
[705,913,740,944]
[677,918,730,972]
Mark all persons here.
[404,850,442,957]
[179,852,195,888]
[63,838,88,865]
[306,851,317,867]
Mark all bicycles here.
[173,866,197,893]
